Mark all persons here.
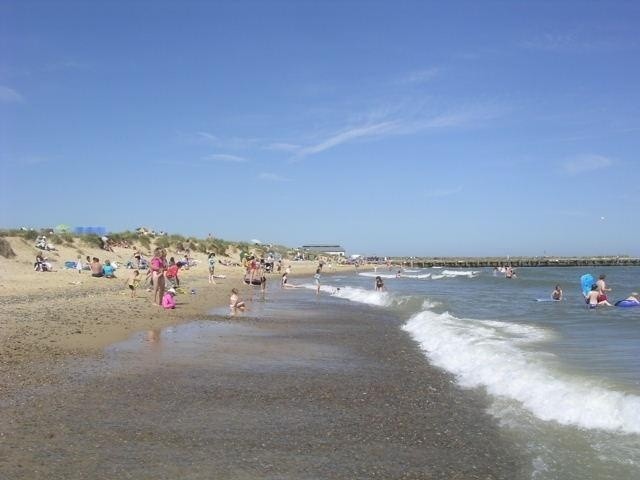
[374,276,385,292]
[550,273,640,309]
[123,245,196,310]
[103,238,130,252]
[34,234,51,251]
[240,248,292,288]
[286,248,393,272]
[491,264,517,280]
[207,252,217,285]
[312,269,321,294]
[34,250,57,272]
[76,255,117,278]
[333,287,341,294]
[230,288,248,311]
[396,270,400,278]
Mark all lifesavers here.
[615,299,638,306]
[581,274,594,292]
